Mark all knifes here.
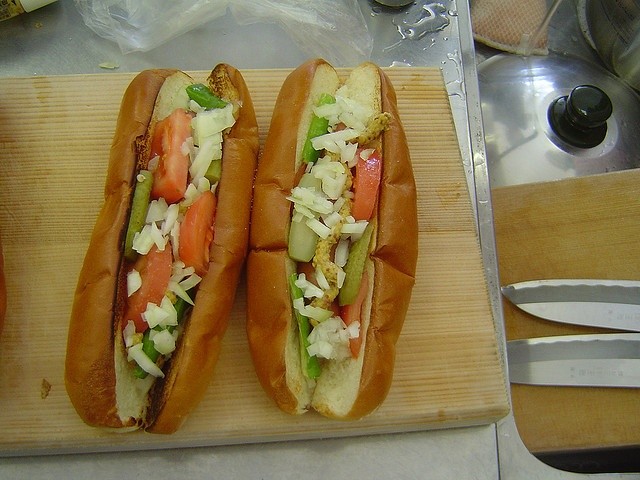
[501,279,640,333]
[506,332,640,388]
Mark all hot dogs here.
[63,66,260,432]
[246,60,420,423]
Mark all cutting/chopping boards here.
[491,168,640,454]
[0,68,511,457]
